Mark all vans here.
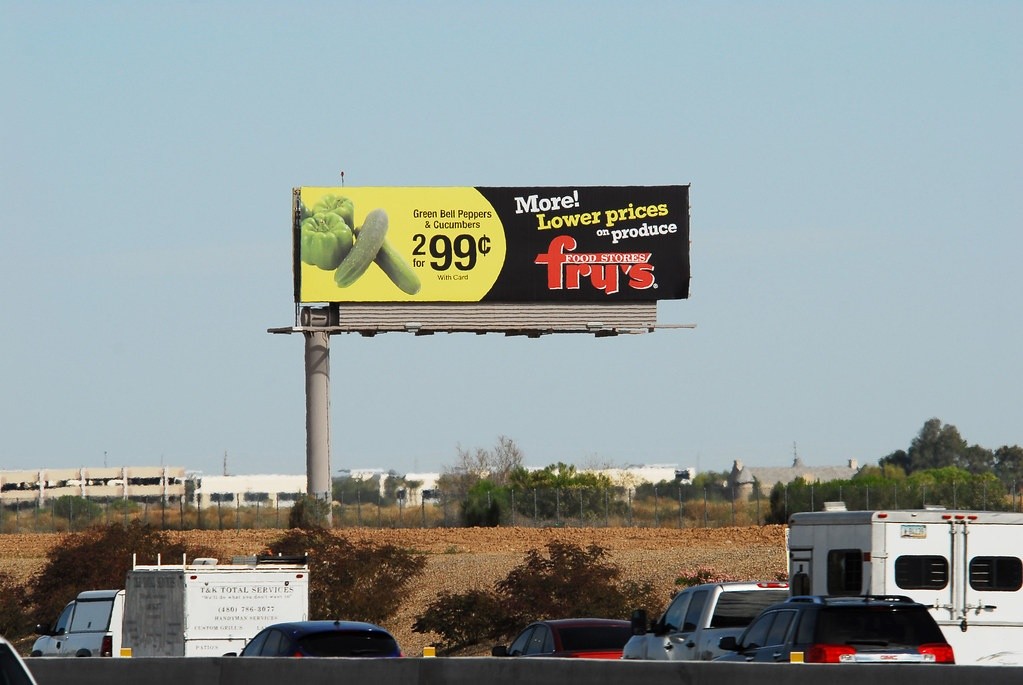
[31,589,128,658]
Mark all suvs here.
[710,575,957,663]
[621,580,789,661]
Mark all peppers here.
[299,195,354,271]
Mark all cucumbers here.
[355,225,421,295]
[333,208,388,287]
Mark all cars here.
[0,636,38,685]
[491,618,632,660]
[238,615,407,658]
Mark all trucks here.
[121,552,310,658]
[788,506,1023,666]
